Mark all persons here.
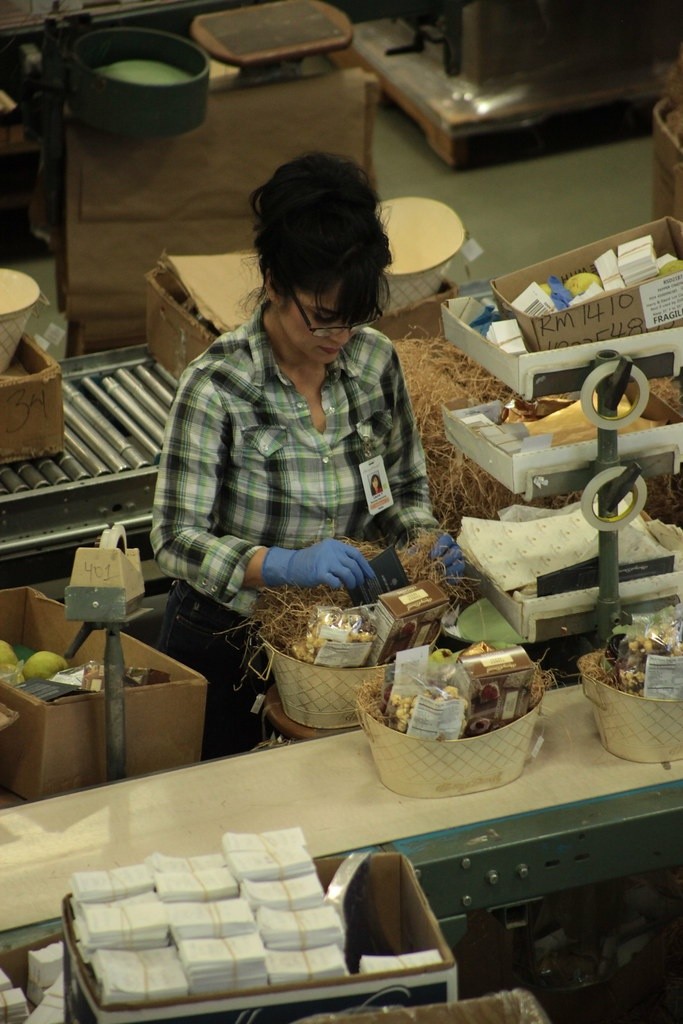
[369,474,383,498]
[149,151,466,761]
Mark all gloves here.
[262,537,375,591]
[408,531,465,584]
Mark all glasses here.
[289,284,383,336]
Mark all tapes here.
[580,359,650,430]
[581,466,647,532]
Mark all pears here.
[538,273,602,298]
[658,260,683,276]
[0,640,69,686]
